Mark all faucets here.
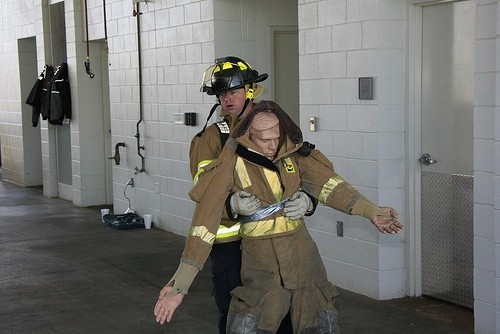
[133,167,141,175]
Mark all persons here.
[189,56,334,334]
[154,99,404,334]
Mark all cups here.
[143,214,152,230]
[100,209,110,223]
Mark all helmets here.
[200,56,268,95]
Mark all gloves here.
[230,191,261,216]
[283,191,313,220]
